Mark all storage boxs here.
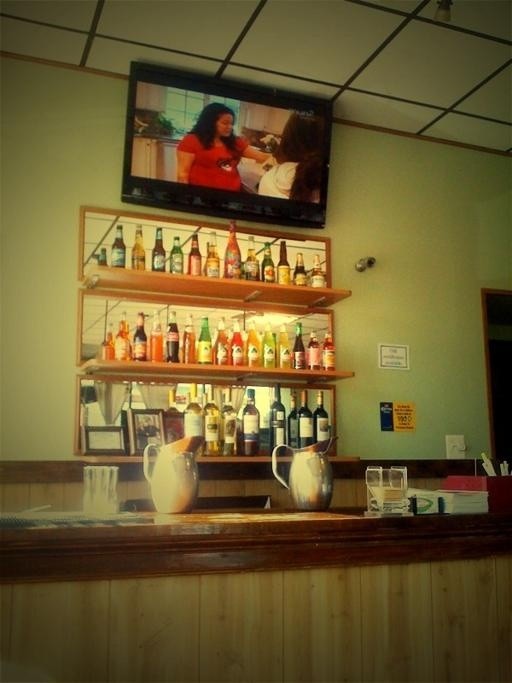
[441,473,512,512]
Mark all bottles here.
[164,382,329,456]
[103,312,337,371]
[111,220,326,289]
[96,247,109,266]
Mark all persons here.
[257,109,325,204]
[176,100,271,192]
[136,421,149,450]
[144,418,160,444]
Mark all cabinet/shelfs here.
[73,203,360,462]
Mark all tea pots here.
[142,431,205,514]
[270,435,340,511]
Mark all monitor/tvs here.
[121,60,333,229]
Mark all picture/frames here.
[81,425,128,456]
[127,408,165,457]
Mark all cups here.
[83,466,119,516]
